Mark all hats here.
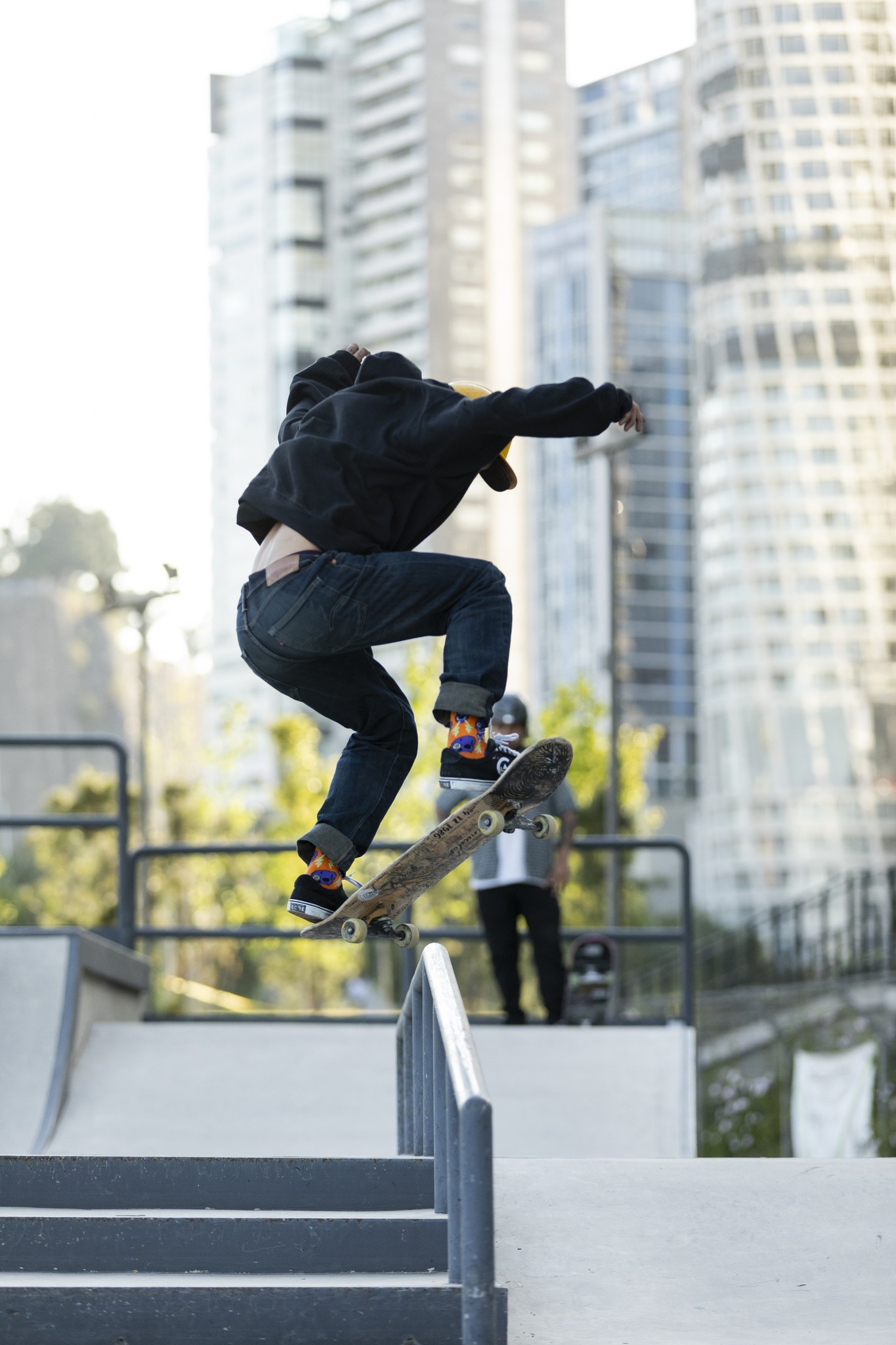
[448,379,518,492]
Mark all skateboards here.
[301,737,573,947]
[566,932,620,1028]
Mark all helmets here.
[493,696,527,727]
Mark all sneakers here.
[286,871,363,923]
[440,731,522,790]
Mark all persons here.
[234,344,647,923]
[465,694,581,1026]
